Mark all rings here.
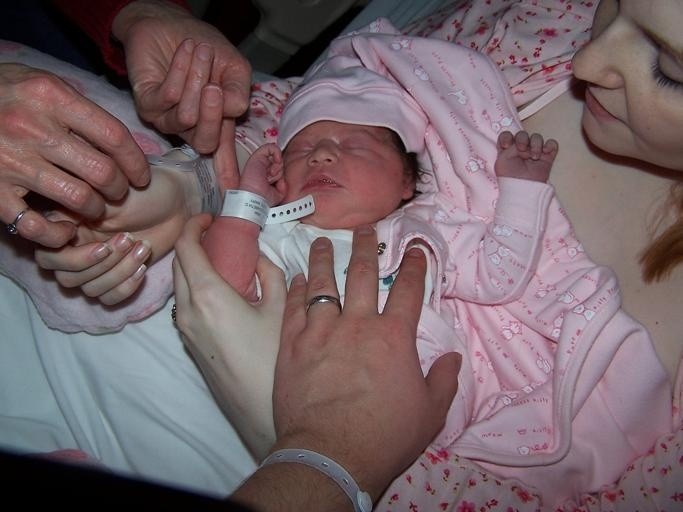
[167,303,181,319]
[301,293,342,320]
[5,204,31,234]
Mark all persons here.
[1,224,470,512]
[0,0,257,254]
[197,59,558,447]
[0,1,680,512]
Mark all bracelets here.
[171,137,222,227]
[256,444,372,512]
[217,187,274,232]
